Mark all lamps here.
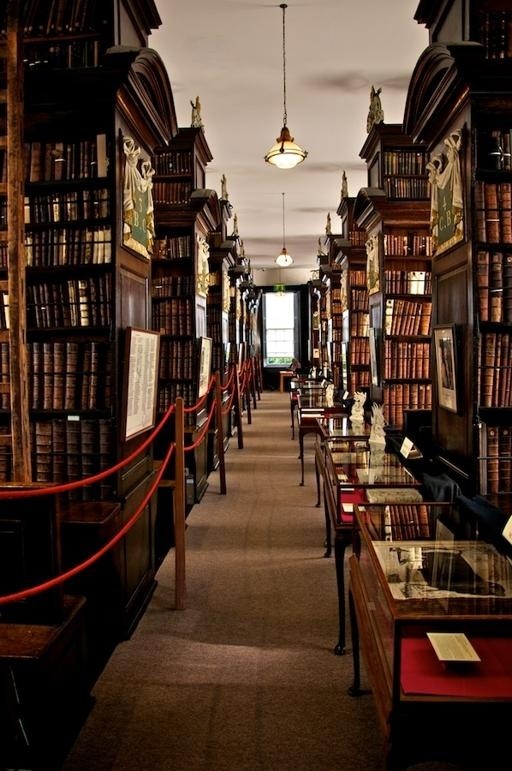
[263,3,308,271]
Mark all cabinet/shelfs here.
[1,1,173,771]
[172,94,263,514]
[304,2,512,545]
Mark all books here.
[383,503,432,543]
